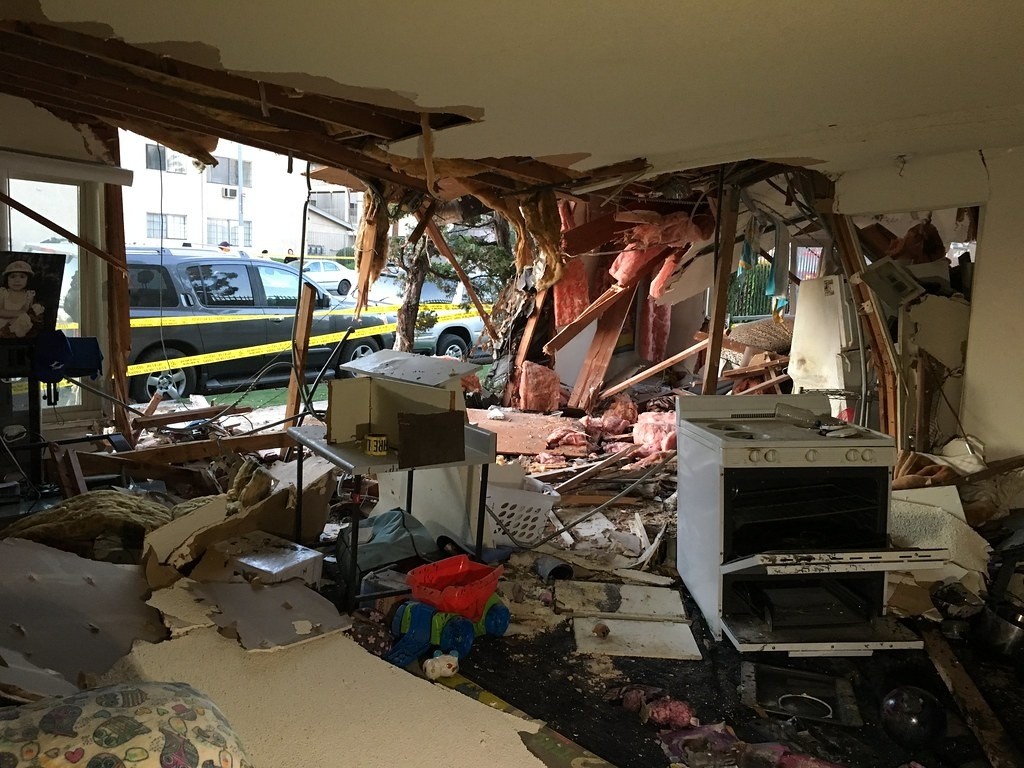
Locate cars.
[282,259,358,296]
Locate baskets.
[483,475,562,543]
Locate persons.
[0,261,42,339]
[262,250,272,261]
[218,242,233,252]
[284,249,298,264]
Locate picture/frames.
[0,251,67,340]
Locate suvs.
[23,241,392,407]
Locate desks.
[289,425,496,603]
